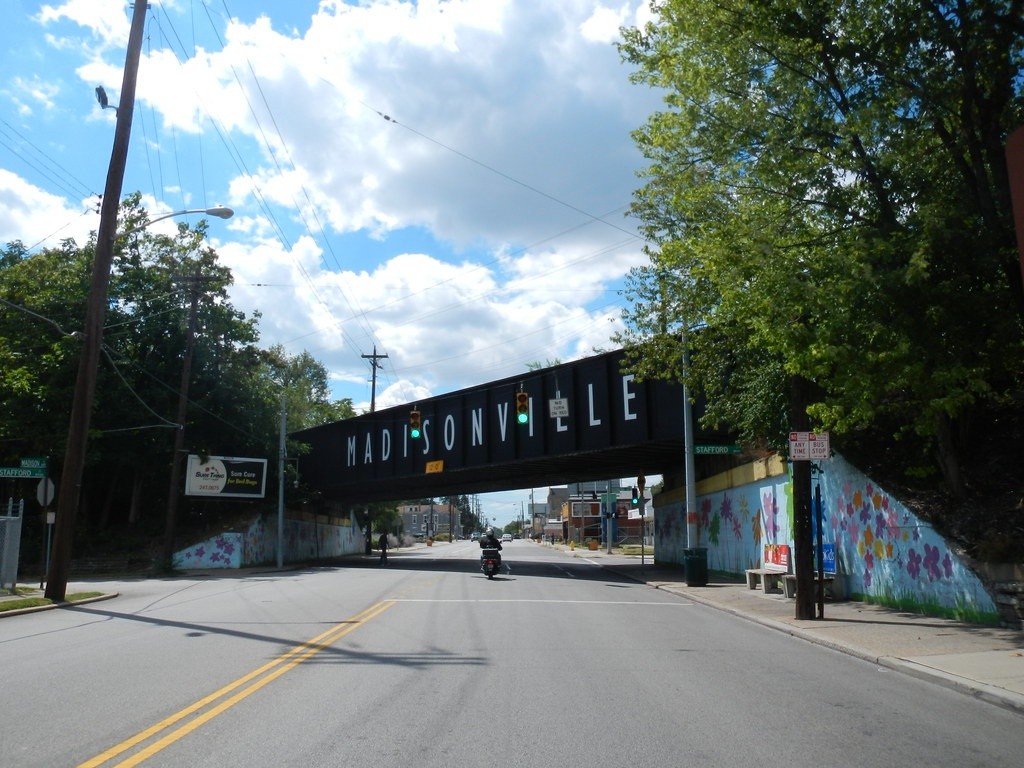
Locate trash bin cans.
[683,548,708,586]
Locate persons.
[480,526,502,570]
[551,532,554,544]
[379,530,390,562]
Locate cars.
[482,533,487,538]
[454,535,465,540]
[471,532,480,542]
[502,534,512,542]
[530,533,544,540]
[514,533,521,539]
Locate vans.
[414,533,427,543]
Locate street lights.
[512,503,525,539]
[43,205,234,602]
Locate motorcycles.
[480,540,502,578]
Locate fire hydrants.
[569,541,575,551]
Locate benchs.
[781,574,836,601]
[745,568,785,593]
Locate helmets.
[486,527,494,535]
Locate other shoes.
[480,563,485,572]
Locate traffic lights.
[518,393,528,425]
[408,410,421,439]
[632,488,639,509]
